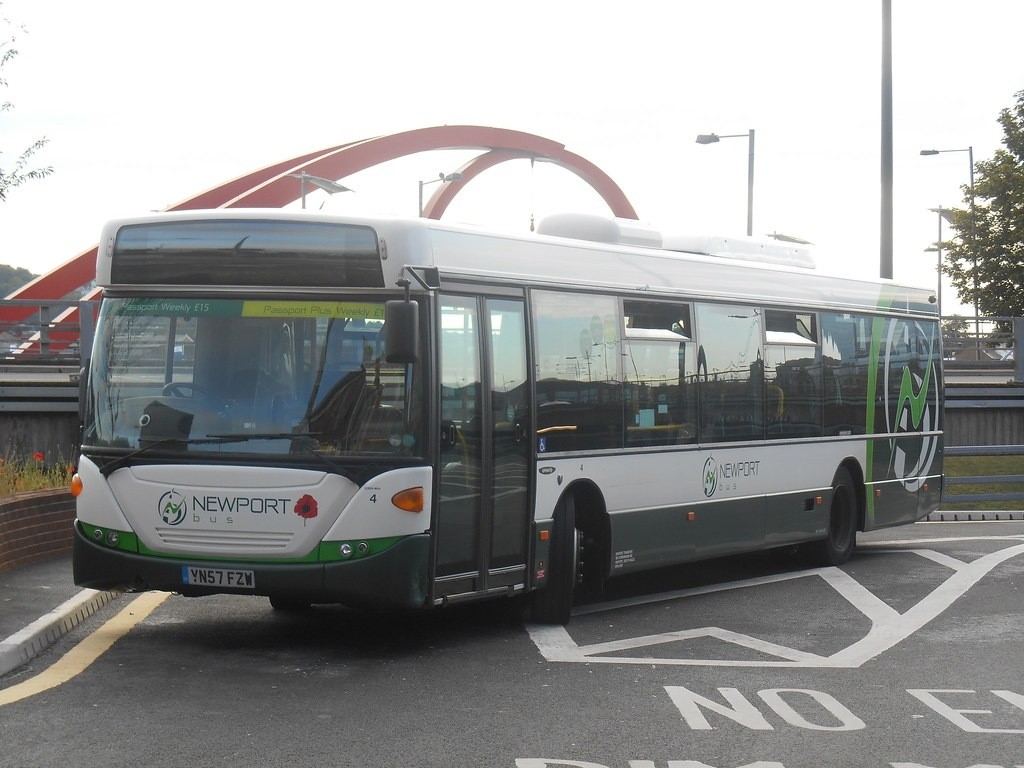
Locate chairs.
[765,366,841,421]
[286,360,366,447]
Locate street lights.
[918,146,981,361]
[696,128,754,237]
[418,171,463,217]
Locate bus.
[70,205,950,629]
